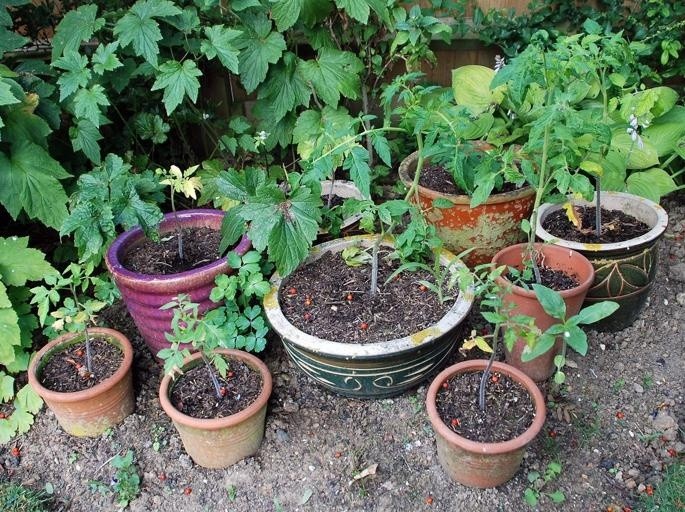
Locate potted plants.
[425,262,548,491]
[26,260,134,440]
[158,291,273,472]
[102,1,599,402]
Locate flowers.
[486,18,665,238]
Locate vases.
[533,190,671,333]
